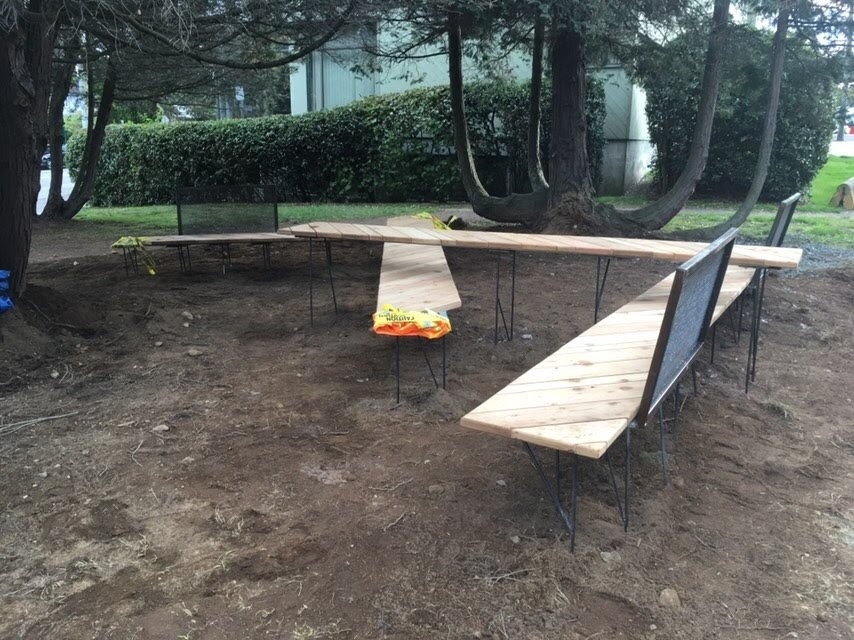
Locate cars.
[41,143,67,170]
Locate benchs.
[111,193,804,552]
